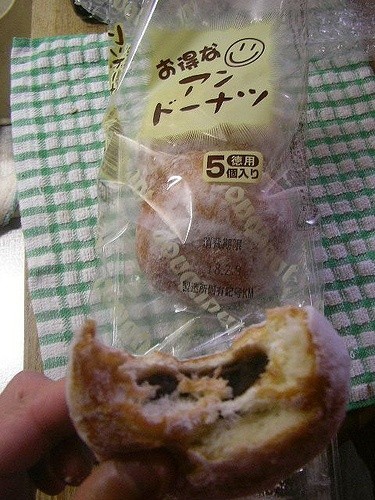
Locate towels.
[10,14,374,412]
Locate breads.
[135,154,295,313]
[61,302,354,494]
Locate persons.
[0,370,174,499]
